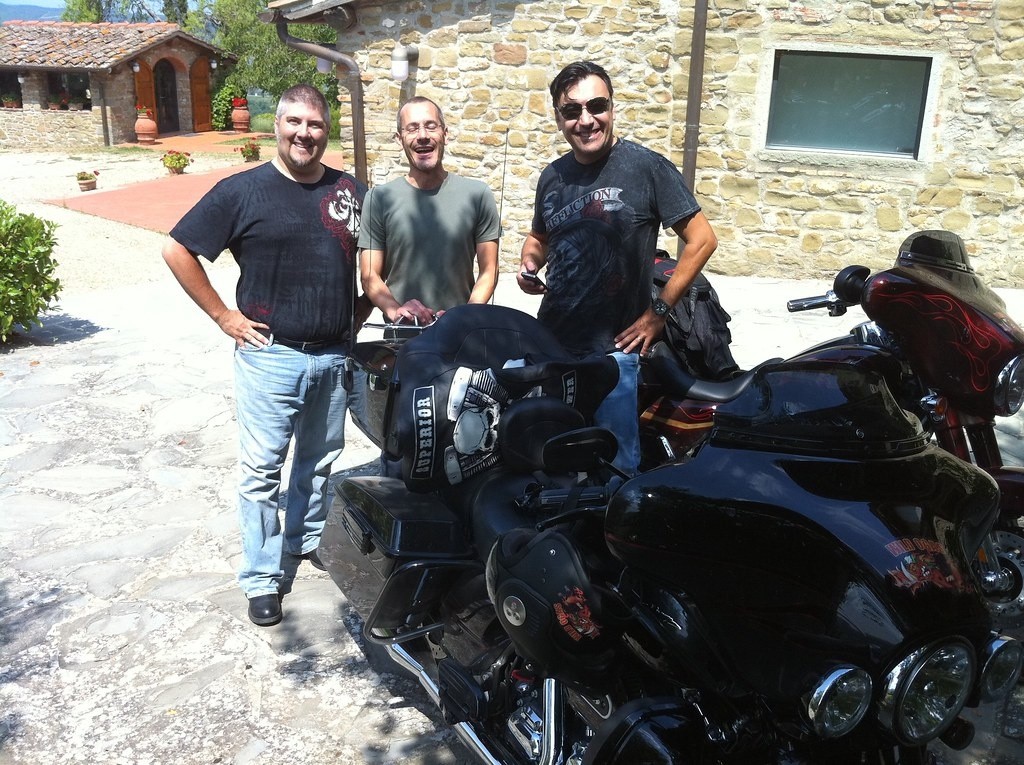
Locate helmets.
[485,528,636,671]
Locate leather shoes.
[292,546,327,570]
[248,594,282,625]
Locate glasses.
[555,95,612,120]
[400,122,443,134]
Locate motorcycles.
[316,230,1023,765]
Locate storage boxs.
[345,335,410,450]
[316,476,466,629]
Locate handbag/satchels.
[647,249,741,382]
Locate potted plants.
[66,96,84,110]
[0,91,22,108]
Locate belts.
[277,337,351,351]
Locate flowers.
[75,171,100,181]
[46,93,70,104]
[233,98,248,107]
[136,105,153,118]
[233,138,260,159]
[160,150,194,168]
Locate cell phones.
[521,272,549,292]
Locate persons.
[516,60,718,481]
[357,96,505,340]
[162,83,369,627]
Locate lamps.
[128,59,140,73]
[208,58,217,70]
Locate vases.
[48,102,62,110]
[231,106,250,134]
[135,113,158,145]
[246,154,259,162]
[167,167,184,174]
[77,180,96,191]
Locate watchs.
[651,298,671,319]
